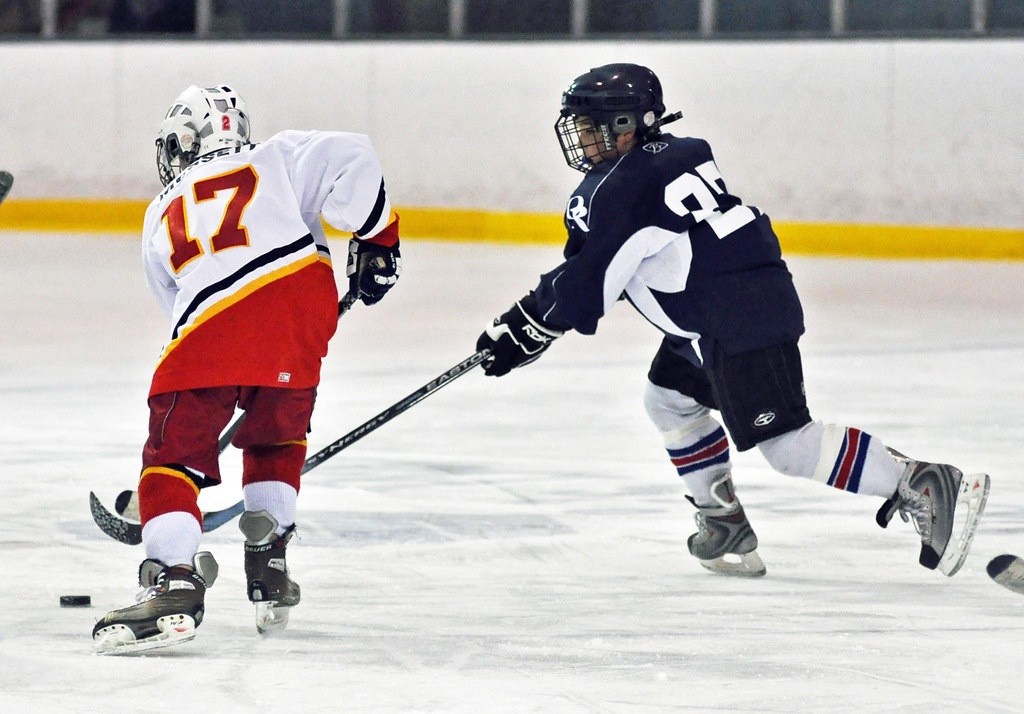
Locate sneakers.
[239,510,300,634]
[93,551,220,654]
[684,492,766,578]
[876,447,989,576]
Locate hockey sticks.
[112,347,493,532]
[985,553,1024,596]
[88,254,387,546]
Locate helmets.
[553,64,664,174]
[155,83,251,185]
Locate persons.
[91,83,402,652]
[476,63,990,578]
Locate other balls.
[59,595,92,609]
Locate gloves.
[477,292,564,377]
[345,238,402,305]
